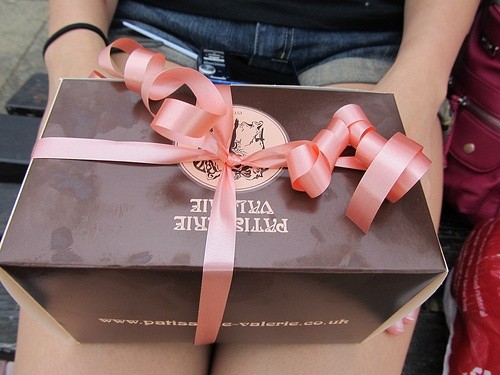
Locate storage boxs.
[0,76,450,346]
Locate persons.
[12,1,481,375]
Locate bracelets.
[41,22,108,58]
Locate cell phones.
[122,19,300,85]
